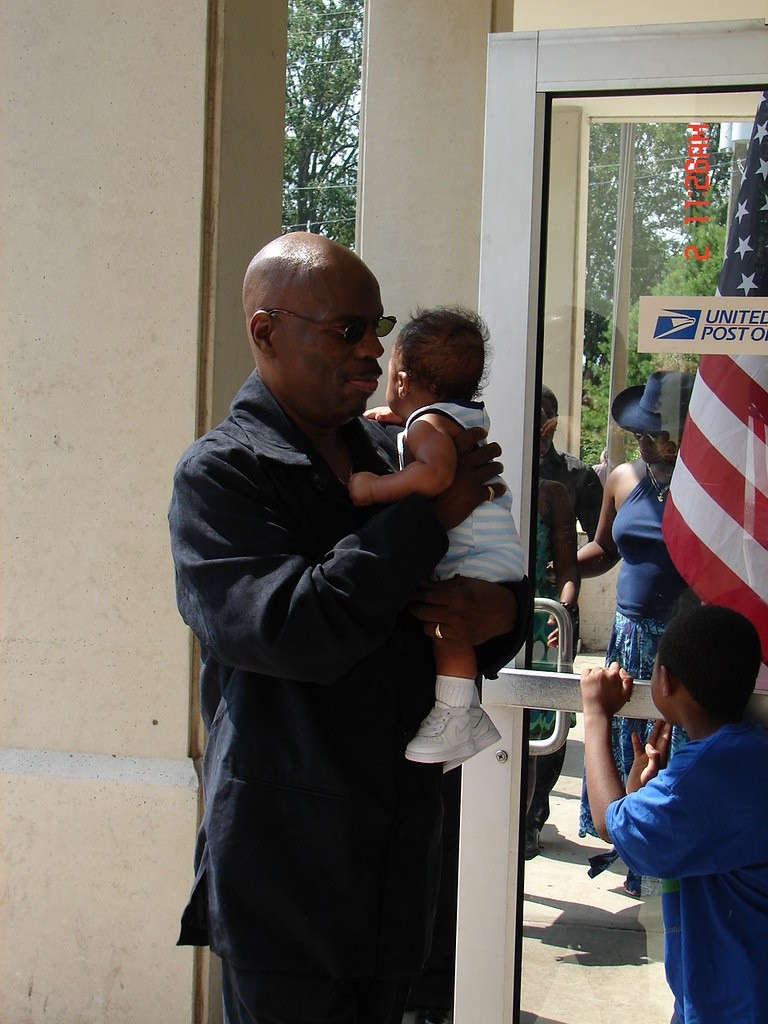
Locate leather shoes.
[526,802,549,860]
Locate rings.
[484,485,494,502]
[434,623,444,639]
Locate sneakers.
[405,707,475,763]
[442,706,502,773]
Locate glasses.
[266,308,396,344]
[636,432,660,441]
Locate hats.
[611,372,695,435]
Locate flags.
[661,91,767,666]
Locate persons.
[581,605,768,1024]
[349,310,524,772]
[522,371,693,895]
[169,233,534,1024]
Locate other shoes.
[625,876,643,896]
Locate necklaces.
[647,463,670,501]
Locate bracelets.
[559,602,578,624]
